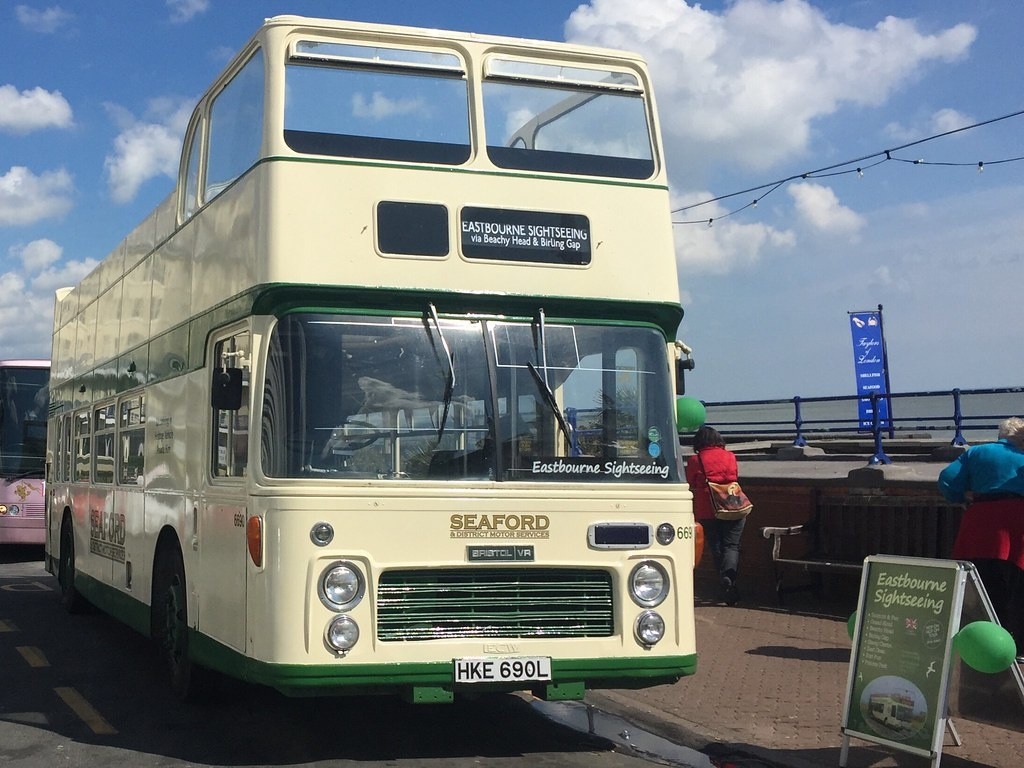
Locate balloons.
[953,620,1016,674]
[847,610,857,639]
[677,397,706,433]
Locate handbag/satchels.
[708,481,753,520]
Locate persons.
[685,426,746,608]
[939,417,1024,656]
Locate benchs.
[759,488,966,606]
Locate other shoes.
[720,570,737,597]
[719,589,744,606]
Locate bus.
[41,12,697,717]
[0,360,50,545]
